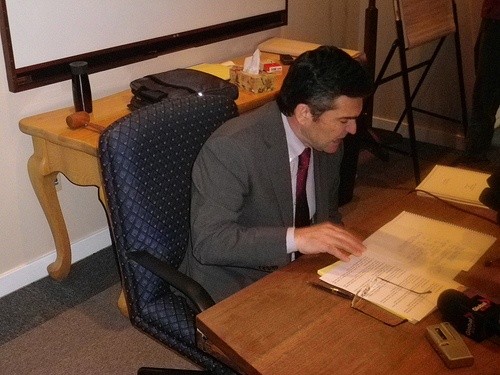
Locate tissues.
[228,48,275,94]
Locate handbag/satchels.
[127,67,239,112]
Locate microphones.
[436,288,500,344]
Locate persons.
[454,0,500,163]
[168,44,371,311]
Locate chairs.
[98,94,239,375]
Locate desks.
[19,48,361,318]
[196,166,500,375]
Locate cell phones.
[279,54,295,65]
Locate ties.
[294,147,311,261]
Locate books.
[415,164,493,210]
